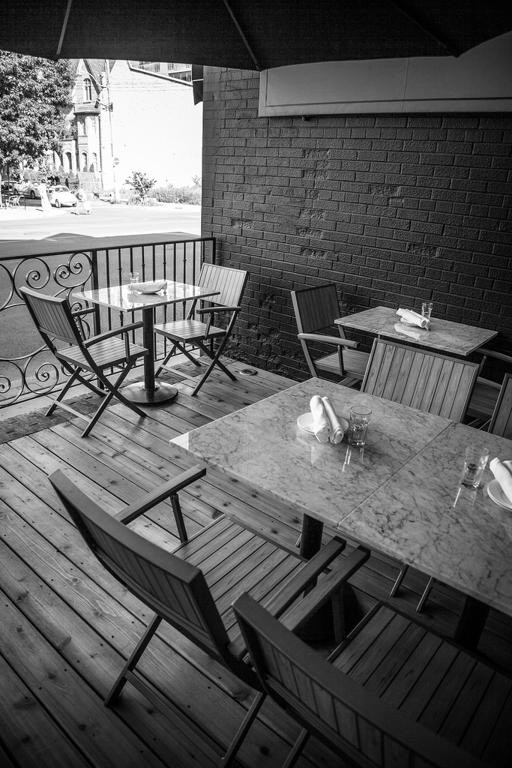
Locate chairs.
[290,283,512,439]
[230,543,512,766]
[48,463,345,768]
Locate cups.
[461,444,488,489]
[128,272,139,290]
[346,407,373,446]
[420,301,432,323]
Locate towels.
[489,457,512,503]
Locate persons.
[38,179,52,213]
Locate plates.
[297,412,348,436]
[486,478,512,510]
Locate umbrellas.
[0,0,511,72]
